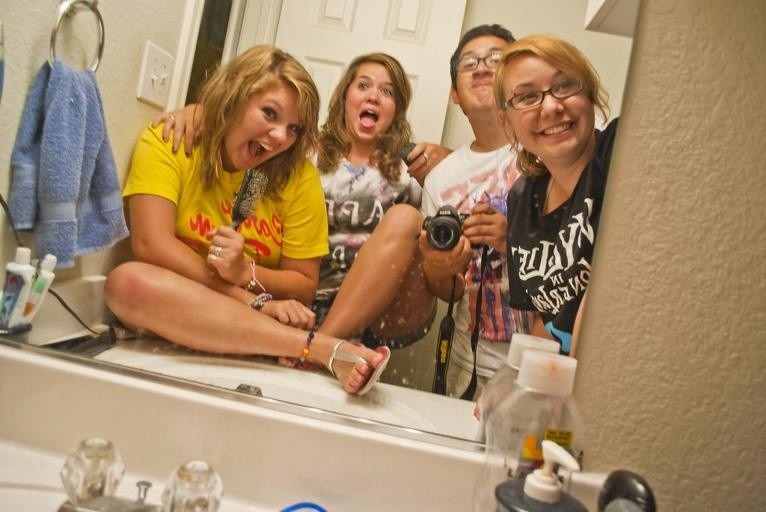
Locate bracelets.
[249,292,272,311]
[246,260,265,293]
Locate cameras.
[423,205,482,251]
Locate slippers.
[329,340,390,396]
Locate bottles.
[473,333,564,446]
[470,351,584,512]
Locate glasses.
[451,54,503,76]
[502,78,582,110]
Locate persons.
[418,24,540,403]
[474,34,620,423]
[105,43,392,398]
[152,53,454,370]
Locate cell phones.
[400,143,423,186]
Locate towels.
[5,58,131,272]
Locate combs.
[232,168,268,232]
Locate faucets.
[235,382,264,396]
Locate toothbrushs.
[24,253,57,316]
[22,270,56,325]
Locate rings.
[168,115,175,122]
[423,153,429,162]
[209,255,216,265]
[214,246,221,257]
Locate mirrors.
[0,0,648,456]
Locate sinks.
[1,482,68,510]
[147,362,435,433]
